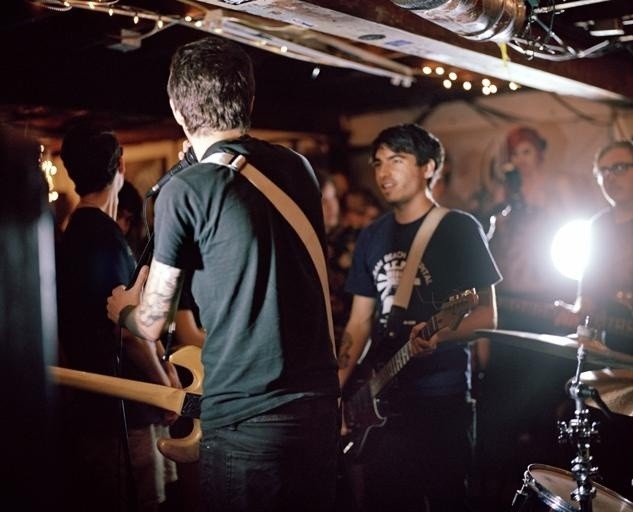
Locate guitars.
[496,291,633,338]
[49,344,205,463]
[338,288,480,466]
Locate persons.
[312,148,471,327]
[117,179,142,239]
[107,37,341,512]
[495,127,571,292]
[336,123,497,512]
[555,142,633,355]
[55,129,183,512]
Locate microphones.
[145,145,199,197]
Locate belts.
[244,395,338,422]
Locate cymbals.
[566,369,633,416]
[475,329,633,369]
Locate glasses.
[595,162,633,176]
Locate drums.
[510,465,633,512]
